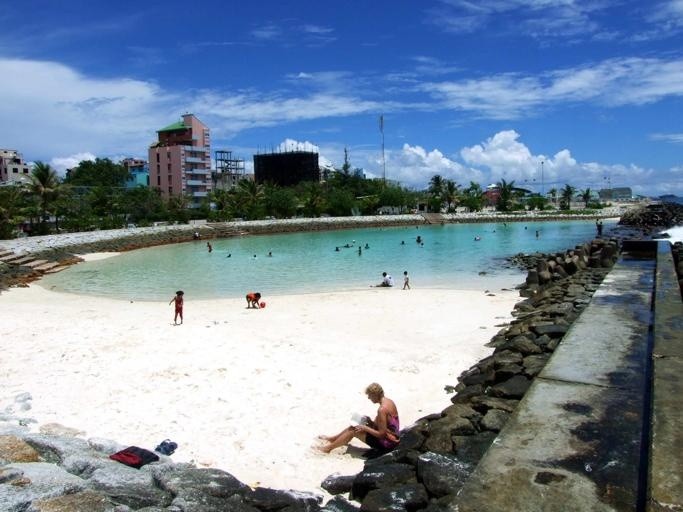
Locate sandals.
[156,439,178,454]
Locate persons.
[246,292,261,308]
[402,271,411,289]
[376,272,394,287]
[335,235,423,255]
[207,242,213,252]
[316,382,399,453]
[169,291,184,324]
[596,218,604,235]
[194,232,199,240]
